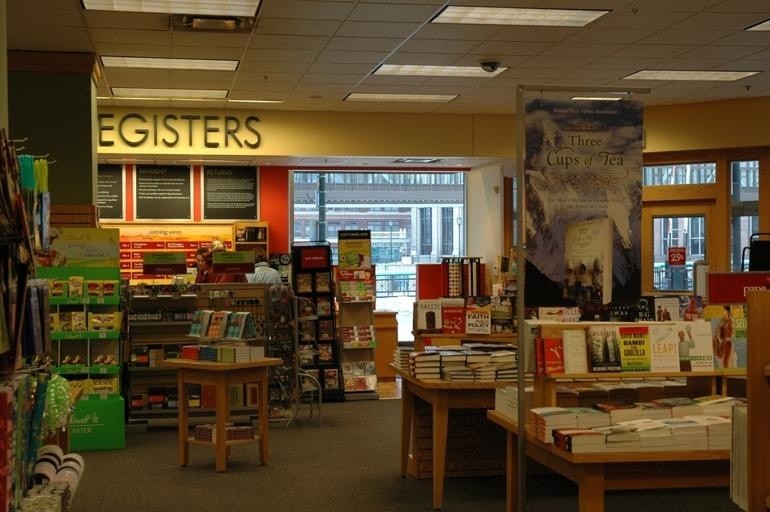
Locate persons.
[681,297,700,321]
[656,306,663,321]
[565,258,604,306]
[662,308,671,321]
[678,325,696,371]
[716,304,736,368]
[190,240,282,283]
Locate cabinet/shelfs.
[33,270,275,475]
[231,221,270,262]
[292,241,378,404]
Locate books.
[552,428,606,454]
[617,419,673,451]
[616,327,650,371]
[703,304,747,368]
[676,321,714,371]
[296,270,339,391]
[589,326,622,372]
[392,341,415,371]
[495,384,519,425]
[439,297,465,333]
[729,405,748,510]
[409,343,518,382]
[693,394,736,417]
[562,329,588,375]
[588,424,641,453]
[491,250,518,315]
[417,299,442,330]
[652,397,703,418]
[678,295,704,321]
[416,257,493,301]
[648,326,680,372]
[653,418,708,450]
[567,408,610,429]
[591,400,641,425]
[629,401,672,419]
[177,309,265,443]
[681,416,731,449]
[523,302,634,335]
[465,295,492,333]
[491,297,515,333]
[630,296,679,321]
[525,405,577,443]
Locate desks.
[386,332,748,512]
[154,352,284,472]
